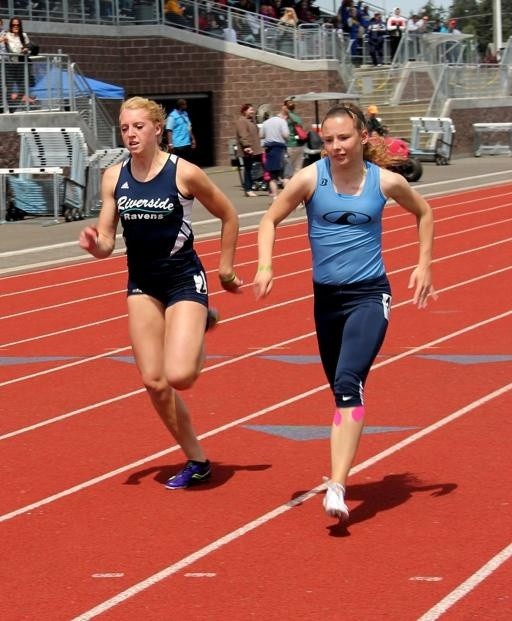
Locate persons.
[237,100,304,197]
[79,96,245,491]
[165,98,195,159]
[3,16,31,112]
[0,1,462,66]
[253,102,439,521]
[366,105,388,137]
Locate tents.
[6,62,124,106]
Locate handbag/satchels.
[292,125,308,140]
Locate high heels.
[245,191,257,197]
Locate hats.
[367,104,380,116]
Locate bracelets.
[222,274,236,283]
[258,264,273,273]
[168,144,173,146]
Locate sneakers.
[321,476,349,519]
[165,457,213,491]
